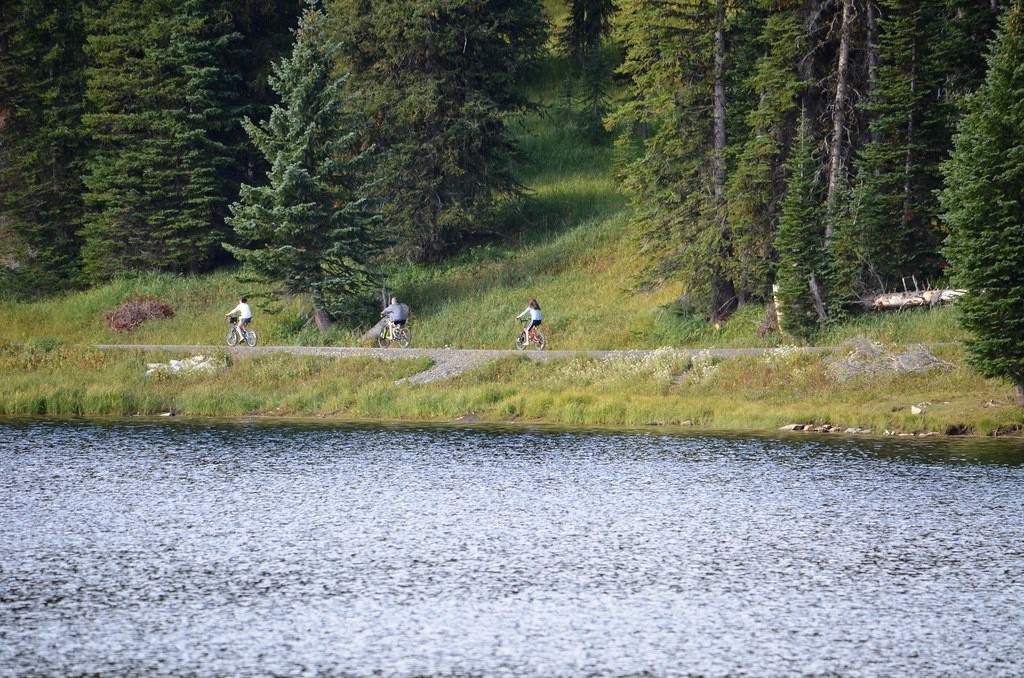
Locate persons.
[381,297,407,341]
[226,296,252,343]
[517,299,541,346]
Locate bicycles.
[516,318,545,350]
[224,315,258,347]
[377,314,412,349]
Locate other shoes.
[240,337,245,341]
[523,342,530,346]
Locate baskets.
[228,317,237,323]
